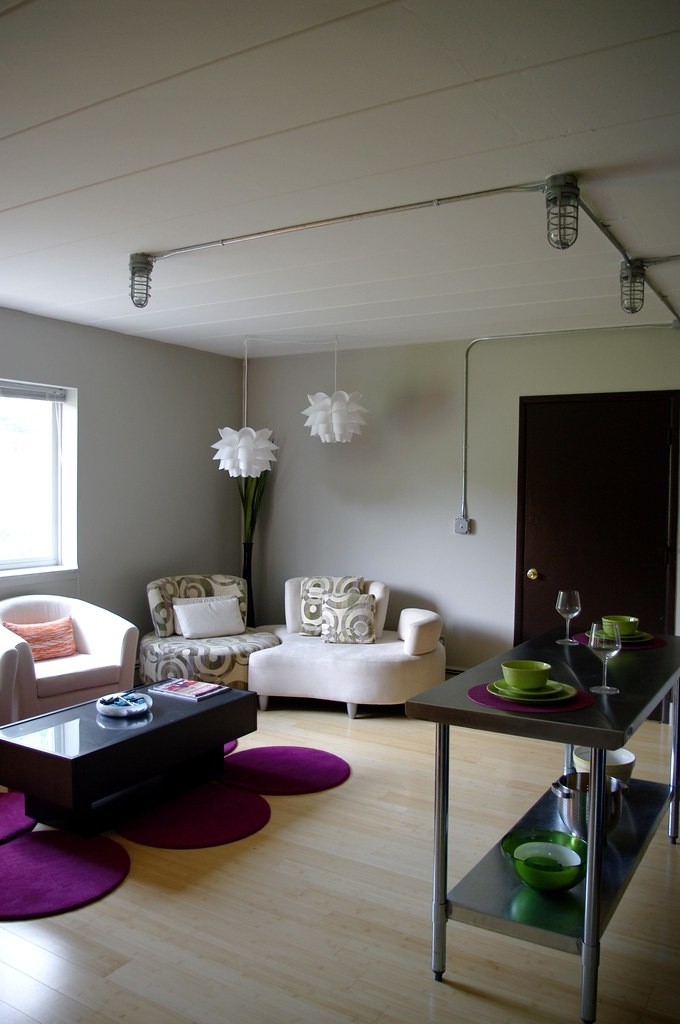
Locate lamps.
[300,337,367,443]
[129,253,153,308]
[545,173,580,249]
[210,338,279,479]
[620,258,645,314]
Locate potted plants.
[235,471,268,628]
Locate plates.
[572,631,672,649]
[467,681,594,712]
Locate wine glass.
[555,590,581,645]
[590,623,621,693]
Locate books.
[148,677,231,703]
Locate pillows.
[321,592,376,644]
[300,575,364,636]
[3,616,78,661]
[172,595,245,639]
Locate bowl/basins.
[501,661,552,688]
[499,883,583,938]
[602,616,639,636]
[500,828,587,892]
[96,692,154,718]
[573,746,636,782]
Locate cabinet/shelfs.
[405,623,680,1022]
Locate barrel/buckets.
[552,772,627,842]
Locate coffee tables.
[0,678,257,835]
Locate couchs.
[139,572,280,718]
[0,594,139,726]
[247,578,447,719]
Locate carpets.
[0,742,350,921]
